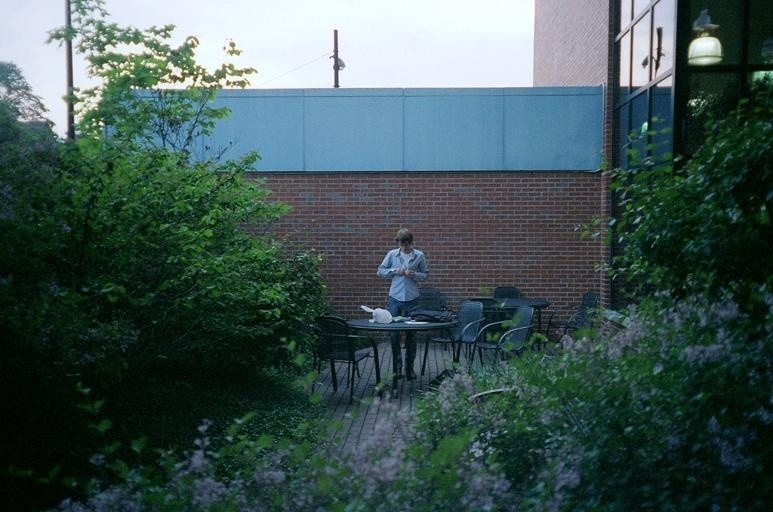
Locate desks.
[347,318,459,399]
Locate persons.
[375,228,429,380]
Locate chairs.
[311,316,370,405]
[416,286,598,372]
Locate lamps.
[687,9,724,66]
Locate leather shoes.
[397,369,416,379]
